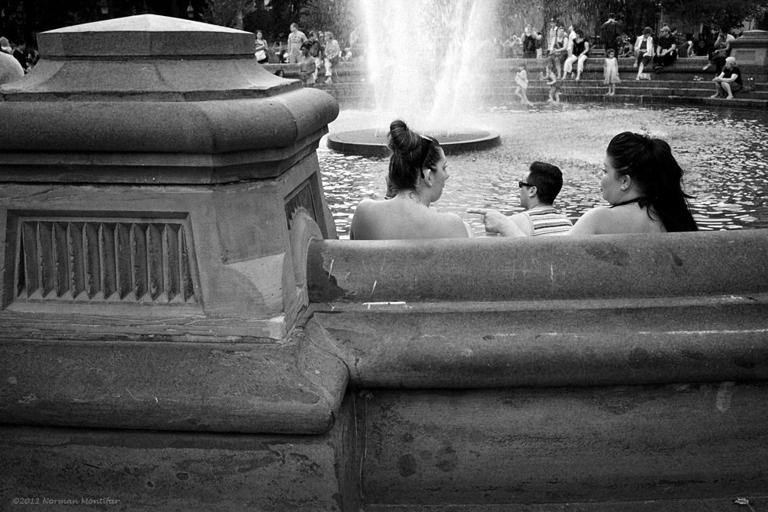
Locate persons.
[497,11,742,106]
[255,22,363,84]
[466,130,699,233]
[497,161,573,238]
[349,120,473,239]
[1,37,38,83]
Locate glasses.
[518,180,528,187]
